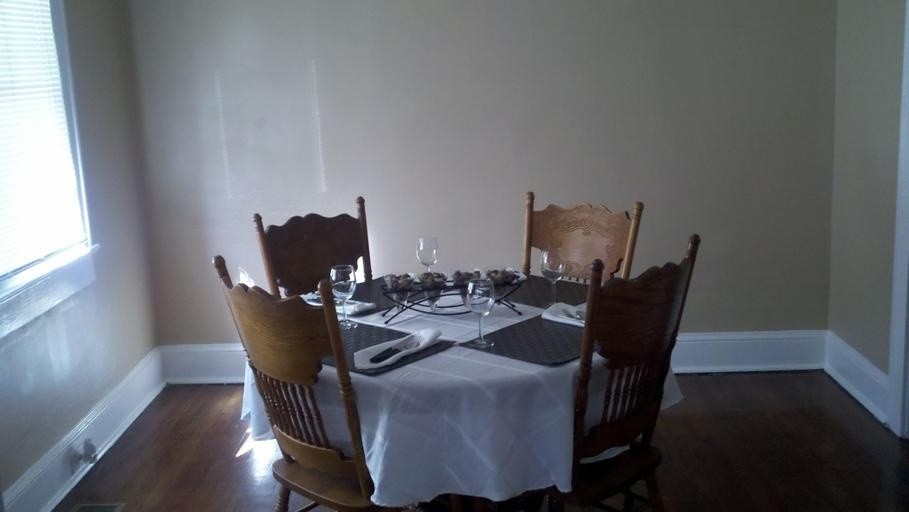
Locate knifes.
[369,334,416,363]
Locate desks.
[275,274,670,512]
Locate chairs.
[515,232,703,512]
[251,196,373,302]
[521,190,645,283]
[211,255,463,512]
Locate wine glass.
[330,264,358,330]
[414,234,440,274]
[465,278,495,349]
[540,250,567,304]
